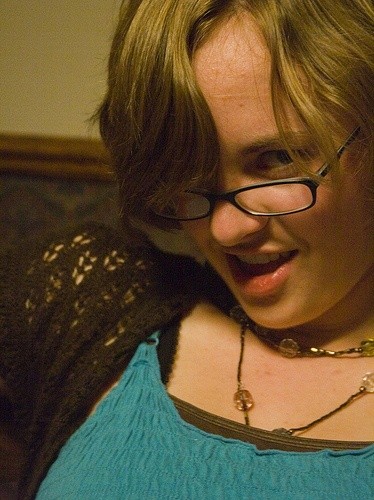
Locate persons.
[0,0,374,500]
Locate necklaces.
[230,302,374,440]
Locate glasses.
[142,115,373,221]
